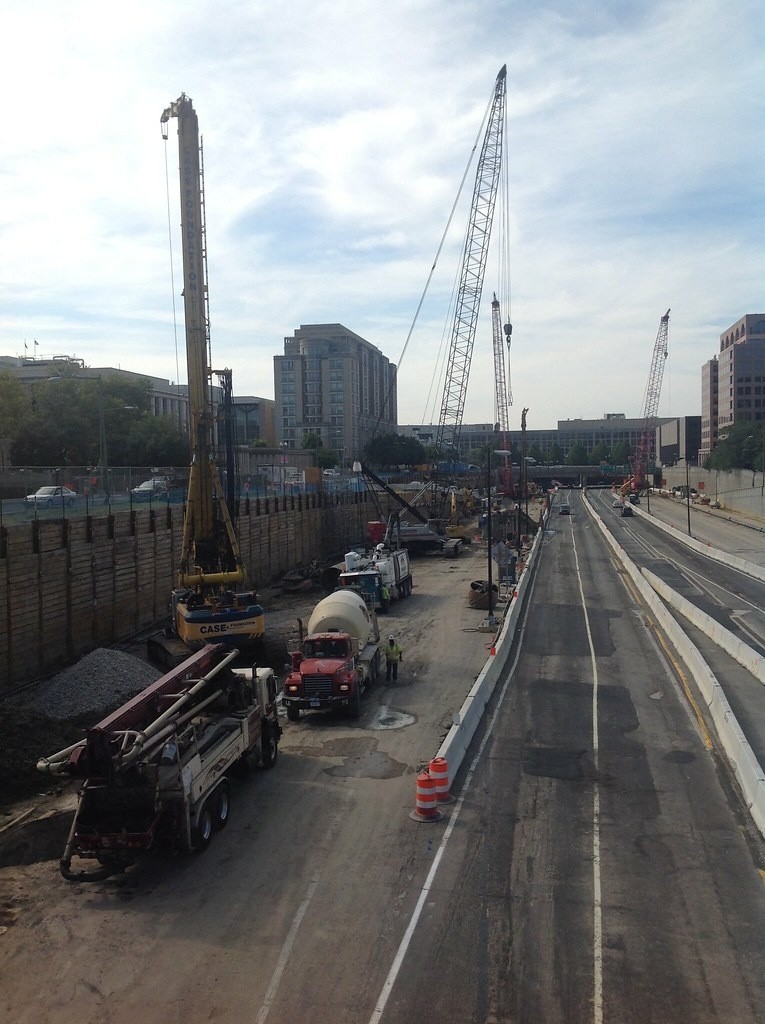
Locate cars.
[129,480,176,501]
[620,507,633,517]
[23,485,77,509]
[629,494,640,504]
[559,505,570,515]
[612,500,624,508]
[242,466,341,497]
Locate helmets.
[389,635,394,640]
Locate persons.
[384,634,403,685]
[379,584,390,614]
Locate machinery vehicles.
[160,90,269,649]
[282,589,380,725]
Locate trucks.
[336,546,413,613]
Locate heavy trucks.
[66,650,285,861]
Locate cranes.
[521,407,531,503]
[490,292,515,502]
[344,61,512,558]
[621,308,672,493]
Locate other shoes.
[385,680,399,685]
